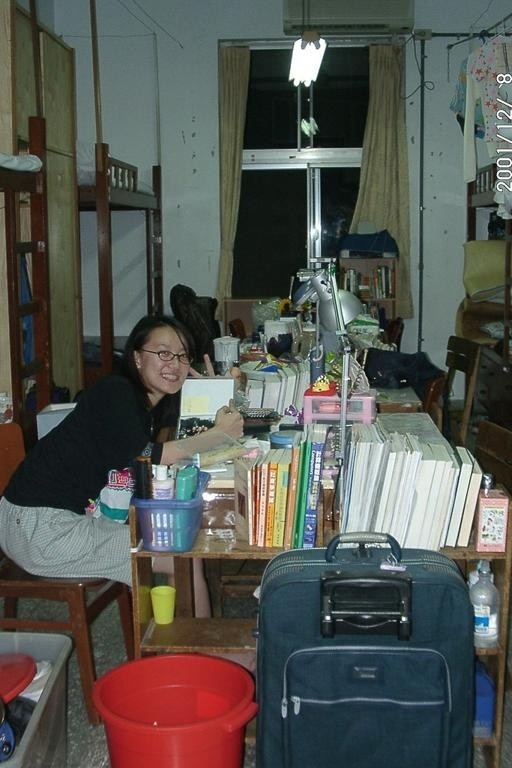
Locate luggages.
[253,532,476,765]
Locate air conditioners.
[283,0,415,36]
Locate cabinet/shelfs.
[0,0,86,398]
[339,256,399,321]
[127,499,512,768]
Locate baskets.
[128,470,211,554]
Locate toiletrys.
[151,464,174,548]
[175,466,198,550]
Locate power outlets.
[414,29,432,40]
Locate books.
[208,417,484,553]
[238,359,312,416]
[174,375,238,440]
[338,263,395,300]
[262,312,306,343]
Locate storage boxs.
[0,632,72,768]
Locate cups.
[150,586,176,625]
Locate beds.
[465,163,512,429]
[77,143,164,403]
[0,117,51,453]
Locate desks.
[173,410,456,619]
[373,383,423,413]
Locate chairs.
[423,368,447,413]
[473,419,512,498]
[441,335,482,448]
[0,423,135,727]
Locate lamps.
[295,115,320,137]
[306,255,364,473]
[288,0,328,88]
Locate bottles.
[469,566,500,648]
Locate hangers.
[479,25,512,47]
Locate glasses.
[142,347,194,365]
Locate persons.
[0,311,258,675]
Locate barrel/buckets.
[94,653,259,767]
